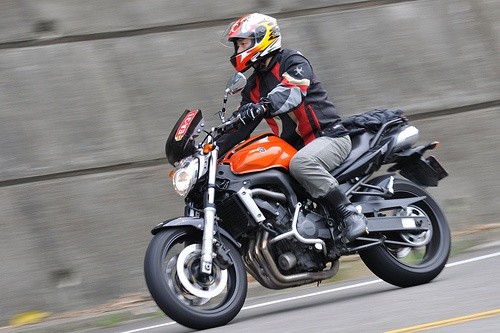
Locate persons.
[216,12,367,242]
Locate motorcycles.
[142,71,453,331]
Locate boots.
[320,185,368,238]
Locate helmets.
[217,11,282,72]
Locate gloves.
[231,103,266,128]
